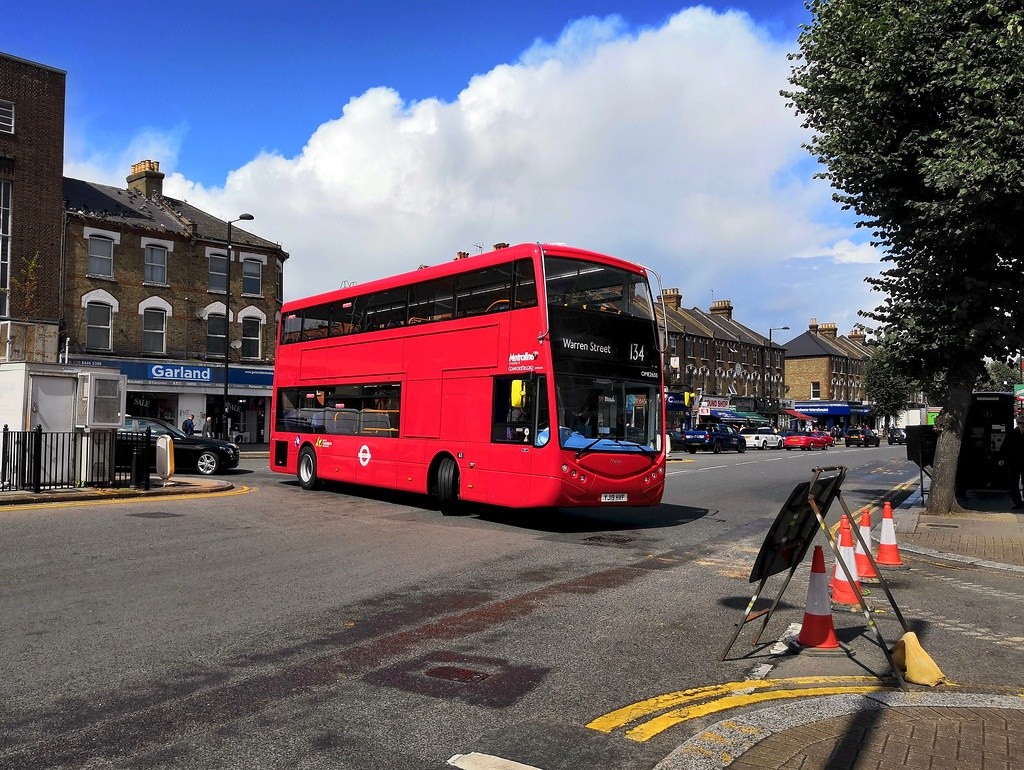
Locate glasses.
[1017,422,1024,425]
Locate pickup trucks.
[681,423,747,454]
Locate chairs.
[723,429,728,433]
[282,318,430,344]
[566,406,591,438]
[285,409,387,436]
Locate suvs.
[844,428,881,448]
[887,428,908,445]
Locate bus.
[269,242,677,517]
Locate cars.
[813,431,836,447]
[785,432,827,451]
[739,428,784,450]
[115,416,241,475]
[655,430,690,454]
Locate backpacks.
[182,419,190,431]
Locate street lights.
[224,212,254,442]
[769,326,791,427]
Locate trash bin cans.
[130,447,146,489]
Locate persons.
[182,414,196,436]
[202,416,213,438]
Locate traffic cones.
[796,545,841,649]
[854,509,877,577]
[832,525,863,605]
[828,514,850,589]
[874,501,903,565]
